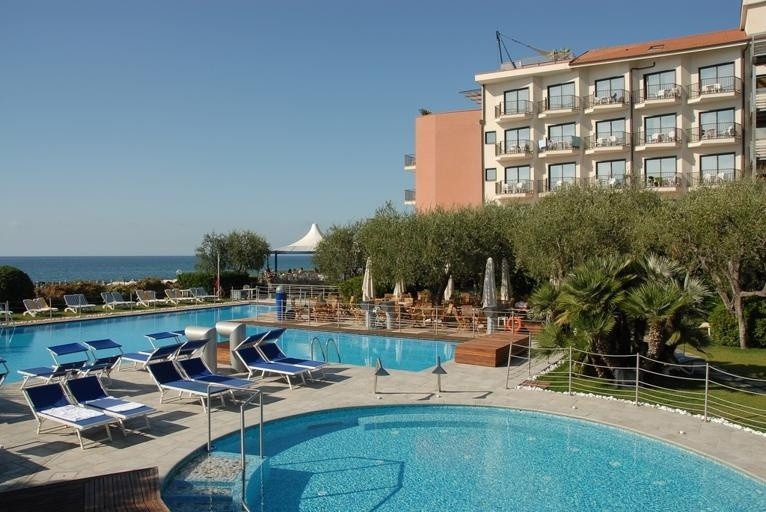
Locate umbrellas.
[362,257,374,302]
[499,256,513,300]
[483,257,497,309]
[444,275,453,302]
[393,277,405,331]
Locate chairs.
[22,286,219,318]
[23,382,119,452]
[258,328,325,383]
[18,343,90,390]
[177,356,257,404]
[147,361,230,413]
[137,329,188,356]
[52,338,122,377]
[122,332,176,370]
[65,375,159,439]
[235,332,309,387]
